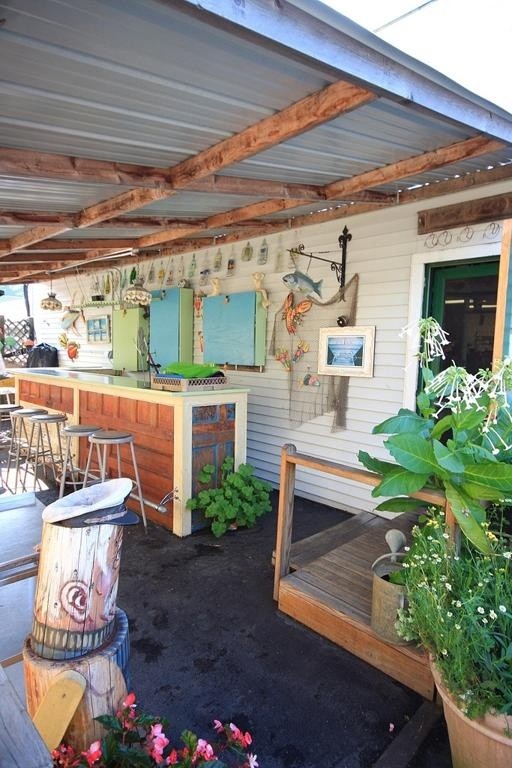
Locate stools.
[0,403,149,536]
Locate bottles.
[89,238,268,294]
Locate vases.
[425,651,509,766]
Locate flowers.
[43,691,261,767]
[405,505,512,722]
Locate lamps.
[38,270,62,311]
[122,260,152,307]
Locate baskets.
[150,372,229,392]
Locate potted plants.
[184,453,273,537]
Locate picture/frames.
[84,315,111,345]
[318,325,376,379]
